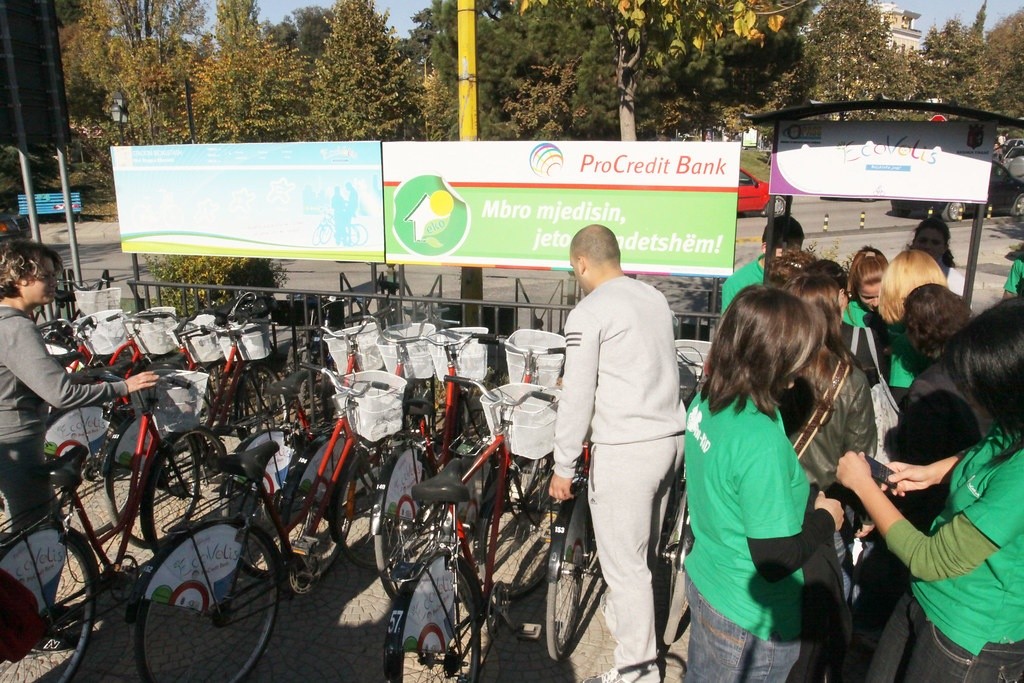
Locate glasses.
[844,291,855,298]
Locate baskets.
[427,327,489,383]
[74,287,121,315]
[333,369,407,442]
[505,329,566,388]
[130,369,210,440]
[165,313,271,363]
[479,382,562,460]
[322,322,437,379]
[124,306,178,355]
[70,309,129,353]
[674,339,714,401]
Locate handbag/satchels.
[850,324,903,465]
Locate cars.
[888,159,1024,220]
[735,169,792,215]
[993,137,1023,184]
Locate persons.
[548,224,686,683]
[0,241,160,653]
[685,217,1024,683]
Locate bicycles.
[0,270,719,683]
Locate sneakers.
[582,661,661,683]
[600,593,620,643]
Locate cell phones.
[864,454,897,489]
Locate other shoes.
[26,603,79,654]
